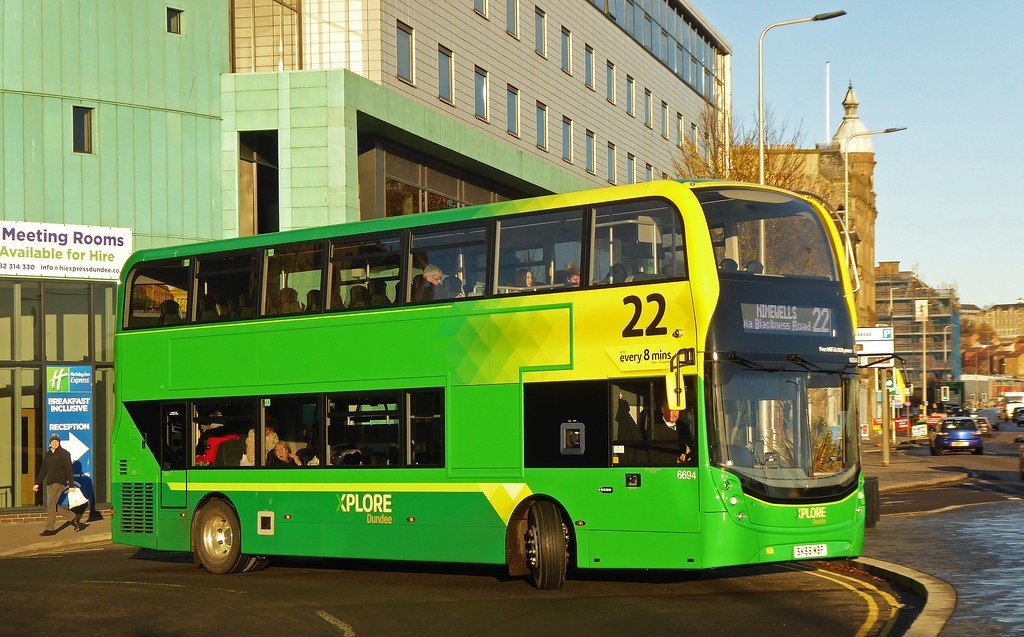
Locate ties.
[670,425,675,431]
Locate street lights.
[943,323,959,363]
[758,8,848,280]
[889,286,910,309]
[845,126,909,269]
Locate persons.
[566,273,580,285]
[32,436,81,536]
[414,264,447,302]
[649,400,693,464]
[196,411,303,466]
[512,269,533,287]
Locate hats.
[50,433,60,443]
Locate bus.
[108,176,916,592]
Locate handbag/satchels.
[68,488,89,509]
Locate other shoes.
[39,529,56,536]
[72,515,81,531]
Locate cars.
[978,409,1000,431]
[1011,406,1024,426]
[974,417,994,438]
[929,416,985,456]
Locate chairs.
[201,430,444,469]
[160,254,763,324]
